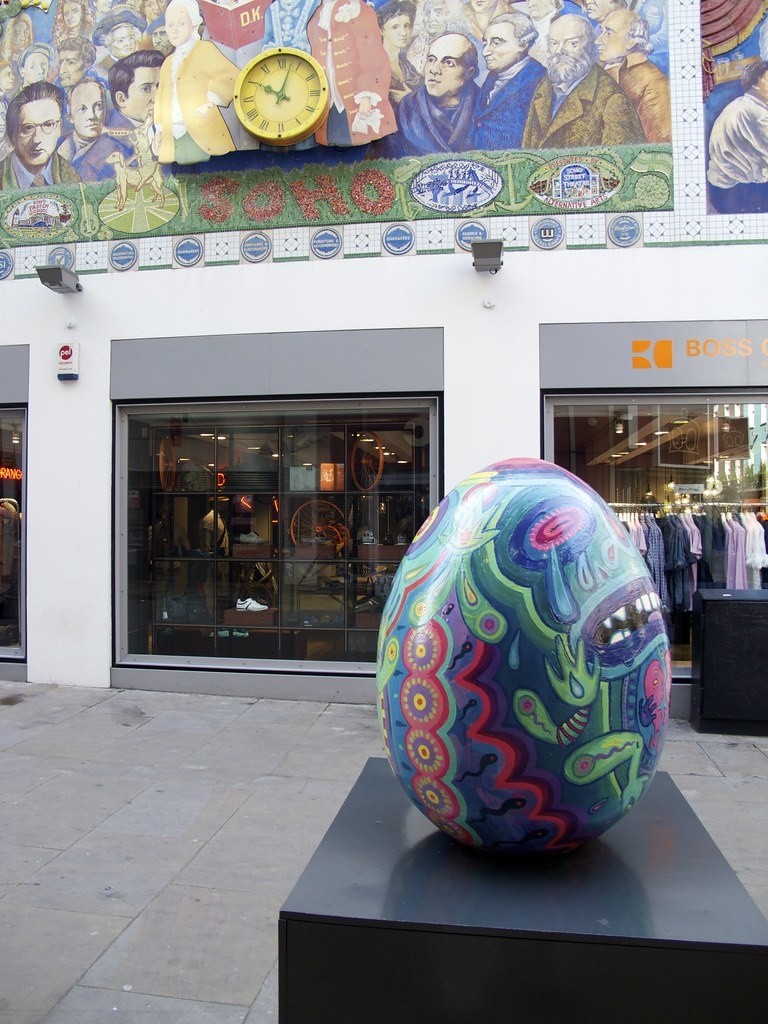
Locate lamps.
[35,265,83,294]
[11,428,19,443]
[721,416,731,433]
[471,240,505,274]
[614,418,624,434]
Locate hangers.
[612,504,766,523]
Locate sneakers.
[287,613,343,626]
[236,598,268,612]
[362,531,377,545]
[392,533,408,546]
[240,531,270,545]
[299,531,331,544]
[352,589,388,614]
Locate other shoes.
[158,545,188,559]
[181,547,214,559]
[0,624,20,645]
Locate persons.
[200,508,230,627]
[641,491,660,513]
[145,502,191,655]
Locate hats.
[208,497,229,502]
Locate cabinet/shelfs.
[130,423,416,657]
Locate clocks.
[234,47,331,146]
